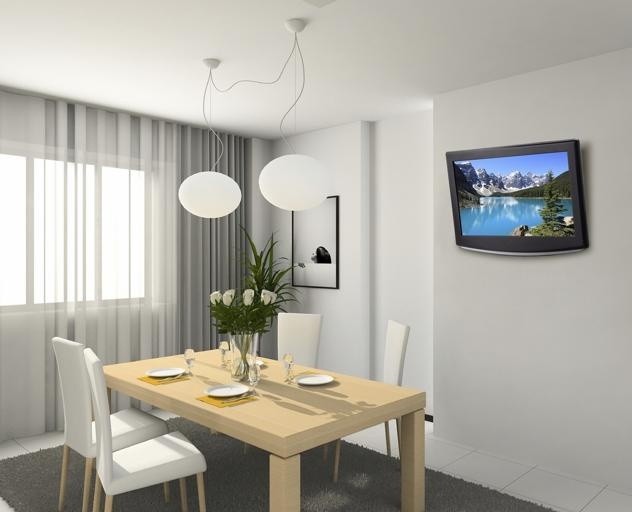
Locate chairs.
[321,318,411,483]
[82,346,208,512]
[242,310,329,458]
[51,336,170,512]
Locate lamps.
[173,17,335,222]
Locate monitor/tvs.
[445,139,589,257]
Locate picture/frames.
[290,195,340,290]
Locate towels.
[299,367,323,380]
[196,394,260,410]
[136,375,191,387]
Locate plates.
[295,375,333,387]
[145,368,185,378]
[204,384,249,399]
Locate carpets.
[0,416,553,512]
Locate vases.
[226,335,263,384]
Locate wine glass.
[184,349,195,377]
[219,341,229,368]
[249,363,260,397]
[283,352,293,383]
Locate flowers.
[205,224,304,382]
